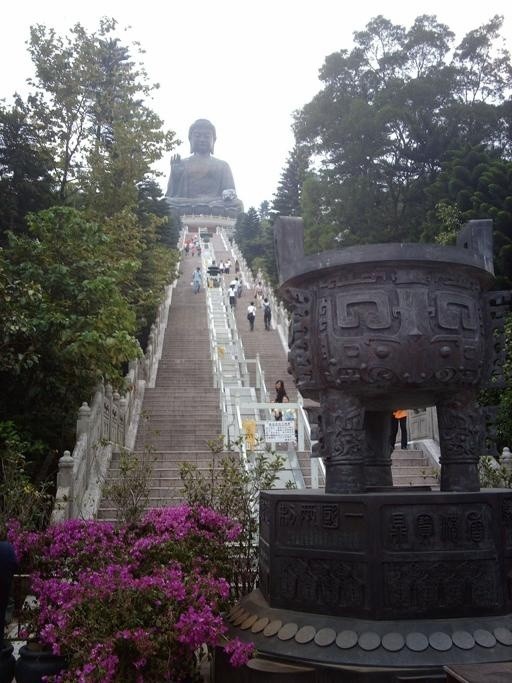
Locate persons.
[253,280,264,308]
[259,295,272,331]
[274,379,286,403]
[390,410,409,450]
[270,395,289,421]
[247,300,257,332]
[219,257,240,274]
[163,118,247,212]
[190,266,202,295]
[228,276,244,311]
[184,232,201,257]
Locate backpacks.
[263,303,271,316]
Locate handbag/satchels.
[247,312,254,320]
